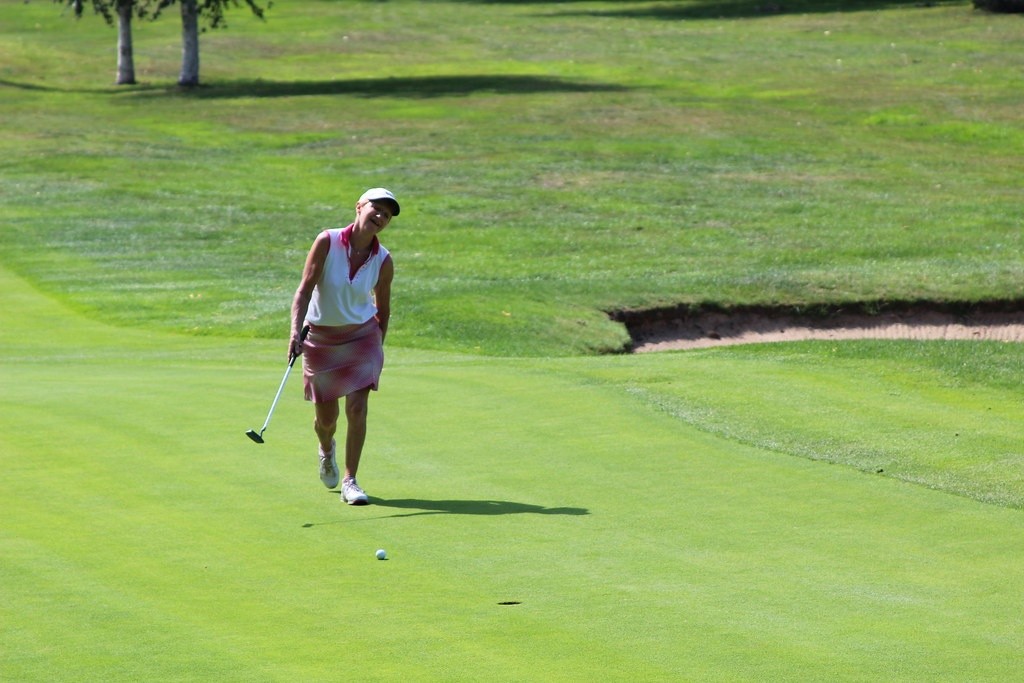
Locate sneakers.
[318,437,340,489]
[339,477,370,506]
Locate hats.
[358,187,401,217]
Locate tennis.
[376,549,386,560]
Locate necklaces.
[357,250,360,256]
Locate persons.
[288,188,400,505]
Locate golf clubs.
[244,324,310,444]
